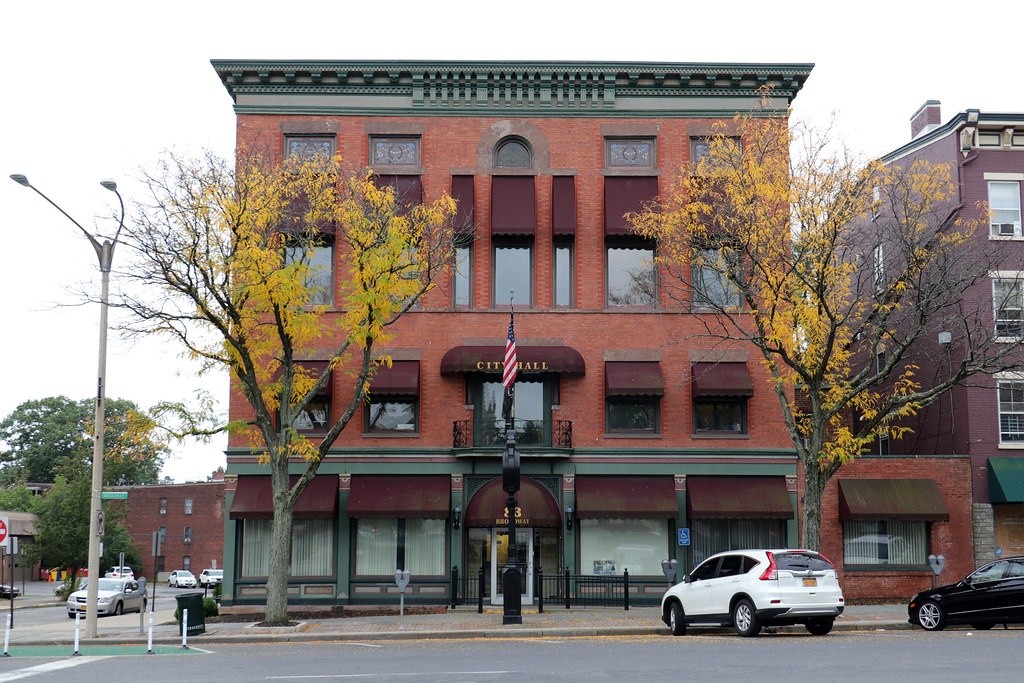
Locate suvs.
[198,569,223,589]
[105,566,135,579]
[660,547,845,637]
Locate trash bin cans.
[174,592,207,637]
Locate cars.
[167,570,197,589]
[55,577,88,597]
[66,577,147,618]
[908,556,1024,631]
[0,584,22,598]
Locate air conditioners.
[186,510,191,514]
[160,510,165,514]
[996,223,1015,236]
[185,538,190,543]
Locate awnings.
[574,475,679,521]
[986,457,1024,503]
[837,479,950,523]
[347,476,451,520]
[229,476,339,520]
[685,475,794,520]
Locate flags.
[502,308,517,390]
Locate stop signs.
[0,517,9,547]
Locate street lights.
[10,173,126,637]
